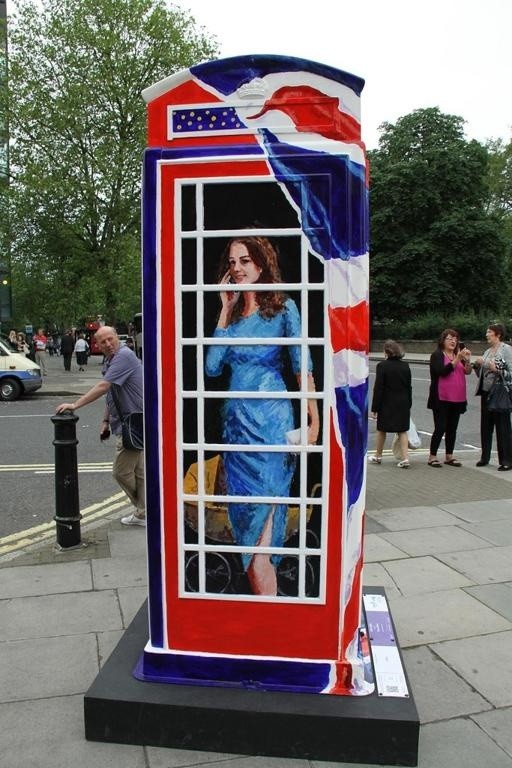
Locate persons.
[9,326,90,376]
[427,329,471,468]
[367,339,411,468]
[473,324,511,471]
[57,326,144,527]
[205,228,319,595]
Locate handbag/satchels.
[121,412,143,451]
[392,434,404,460]
[487,383,512,411]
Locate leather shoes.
[498,465,512,471]
[477,461,488,466]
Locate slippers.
[428,459,442,467]
[445,458,462,467]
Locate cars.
[0,338,42,401]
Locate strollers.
[183,454,322,596]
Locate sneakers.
[121,513,147,527]
[398,459,410,467]
[368,455,382,464]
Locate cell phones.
[459,342,465,351]
[101,430,110,441]
